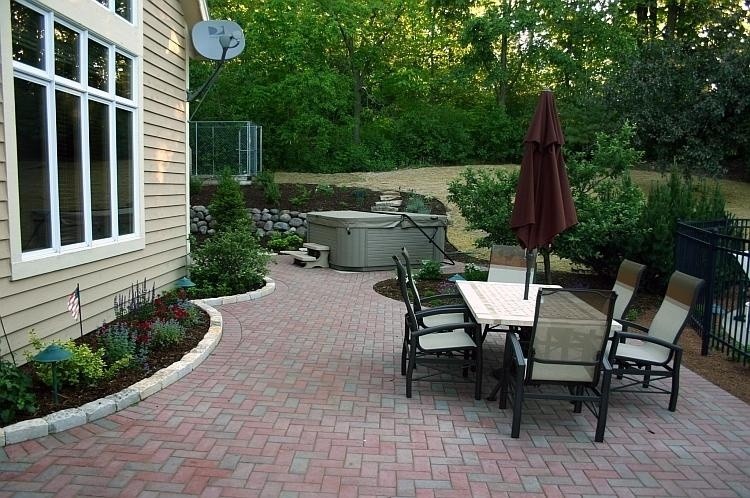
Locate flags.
[67,285,80,323]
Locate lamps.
[32,342,72,400]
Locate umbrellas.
[510,88,581,302]
